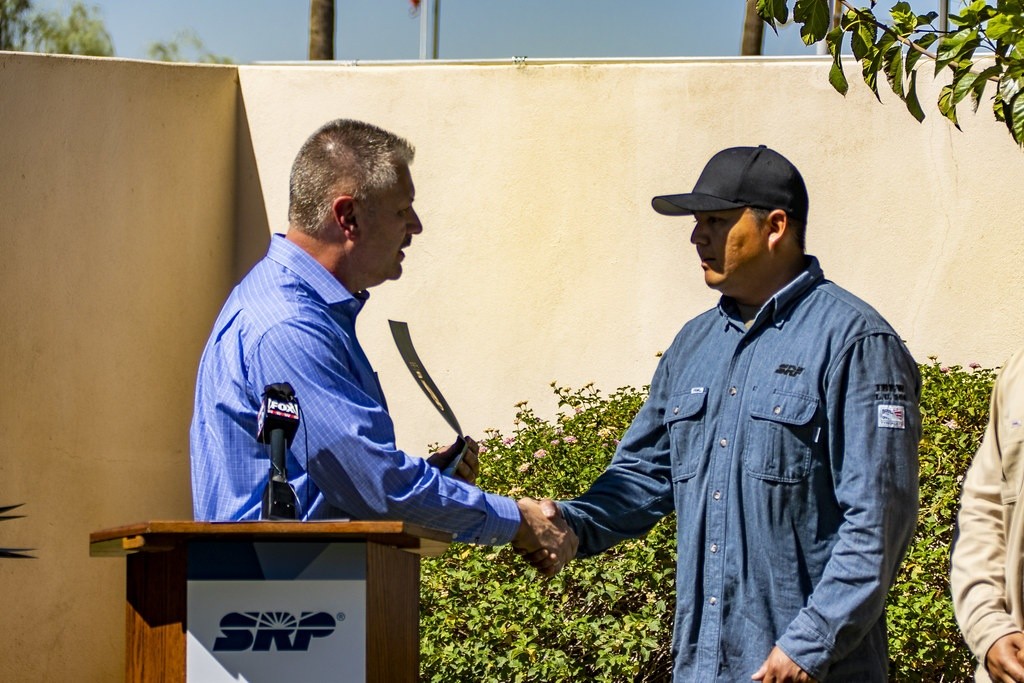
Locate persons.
[188,119,582,579]
[949,344,1024,682]
[512,141,921,681]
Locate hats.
[652,145,814,223]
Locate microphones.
[255,382,300,522]
